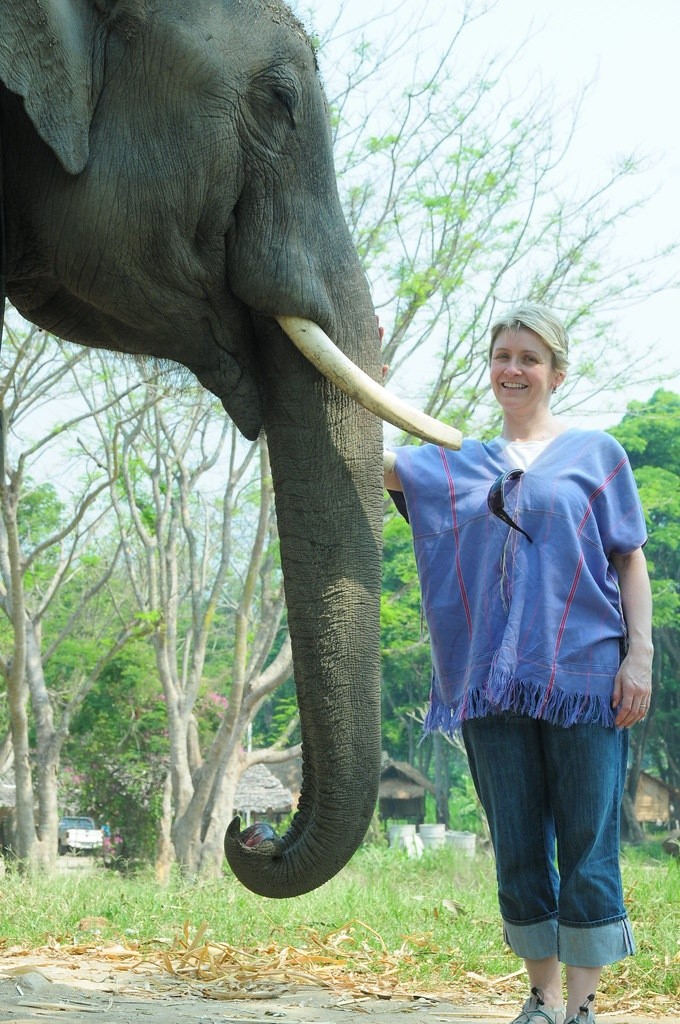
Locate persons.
[375,305,654,1024]
[100,821,124,854]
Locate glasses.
[486,468,533,545]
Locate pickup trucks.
[58,815,104,857]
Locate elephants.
[0,0,465,904]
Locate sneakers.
[561,994,597,1024]
[508,986,567,1024]
